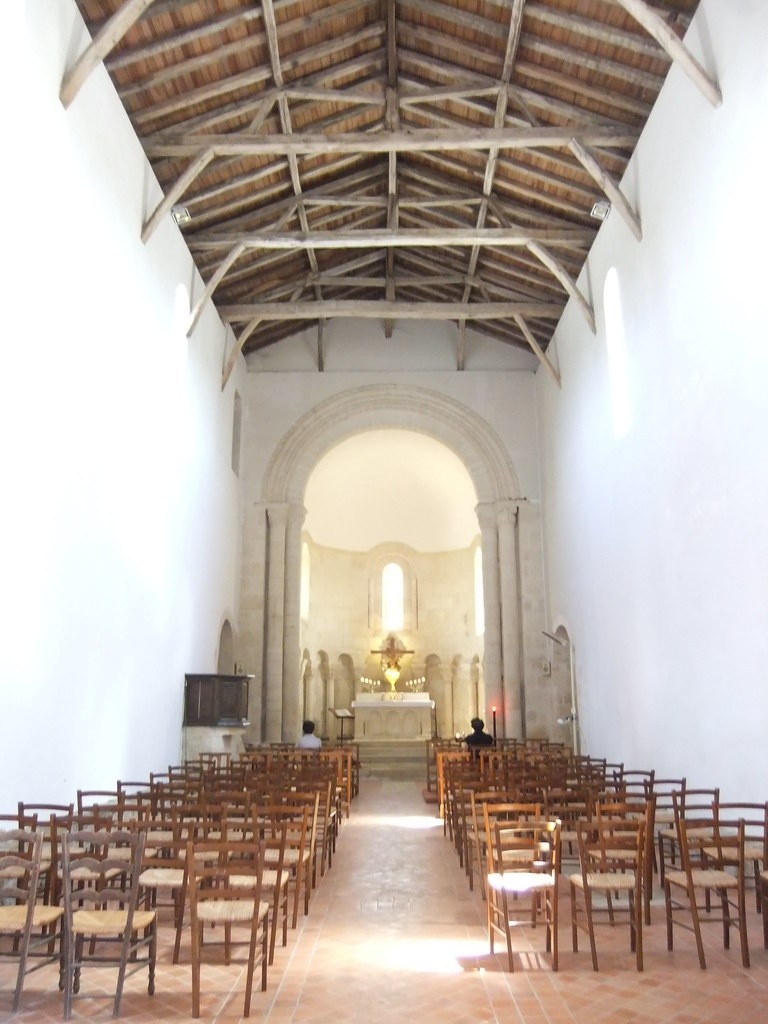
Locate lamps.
[590,200,612,221]
[171,205,192,225]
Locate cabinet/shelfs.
[182,673,255,727]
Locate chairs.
[0,740,365,1018]
[425,737,768,974]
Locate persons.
[295,720,322,749]
[464,718,494,761]
